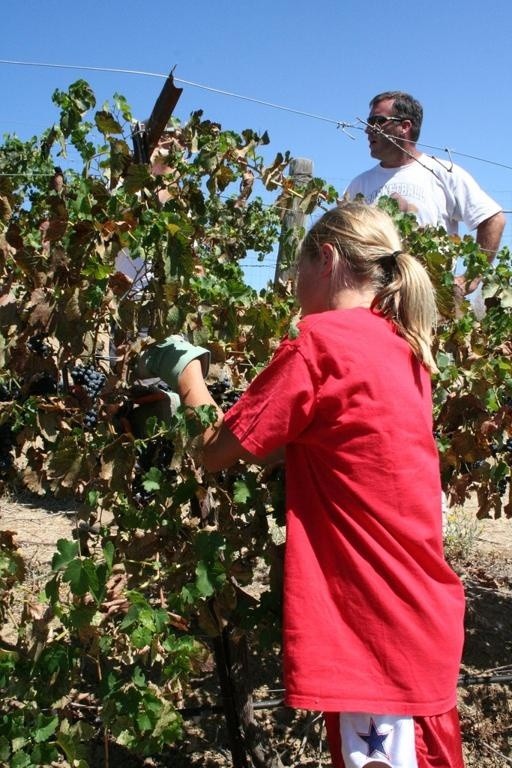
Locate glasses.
[367,115,401,125]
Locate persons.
[102,121,210,500]
[138,203,467,768]
[344,91,506,329]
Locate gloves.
[129,334,210,395]
